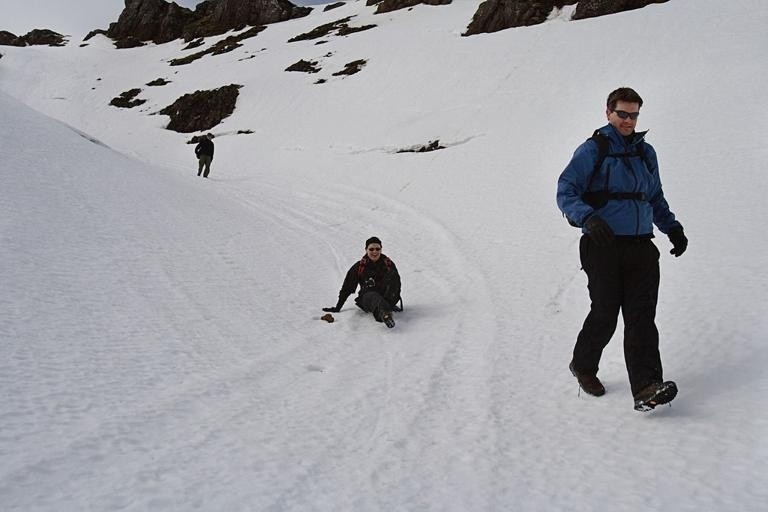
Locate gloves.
[670,235,688,257]
[322,307,340,313]
[586,215,616,248]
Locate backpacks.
[566,135,652,227]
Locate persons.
[195,133,215,179]
[557,88,688,413]
[322,237,401,330]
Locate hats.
[366,237,381,248]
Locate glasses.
[369,248,381,251]
[614,110,639,120]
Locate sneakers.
[570,360,605,396]
[634,381,677,411]
[383,314,395,328]
